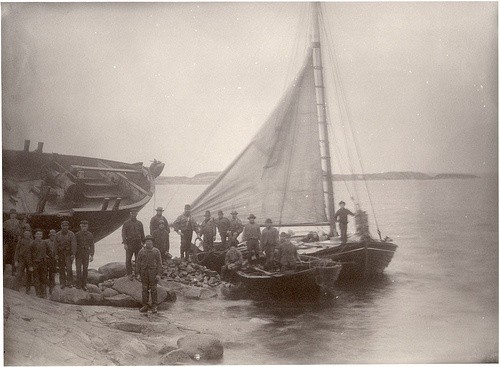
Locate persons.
[261,218,280,258]
[121,208,146,279]
[32,229,48,298]
[55,220,77,290]
[199,210,216,248]
[230,211,244,245]
[150,206,169,257]
[135,235,162,313]
[15,224,32,295]
[174,204,199,261]
[280,234,299,270]
[221,244,244,288]
[74,219,95,290]
[3,208,22,294]
[45,229,56,287]
[244,213,263,266]
[216,210,230,243]
[333,200,355,242]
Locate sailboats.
[171,2,398,280]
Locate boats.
[220,254,343,299]
[186,240,238,272]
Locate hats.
[155,206,164,211]
[158,219,165,225]
[185,204,191,211]
[231,210,237,214]
[143,234,155,241]
[218,211,223,215]
[280,232,285,236]
[129,208,138,211]
[48,229,56,236]
[284,234,291,238]
[265,218,272,222]
[247,214,256,220]
[339,201,345,204]
[204,210,210,216]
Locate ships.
[2,139,165,265]
